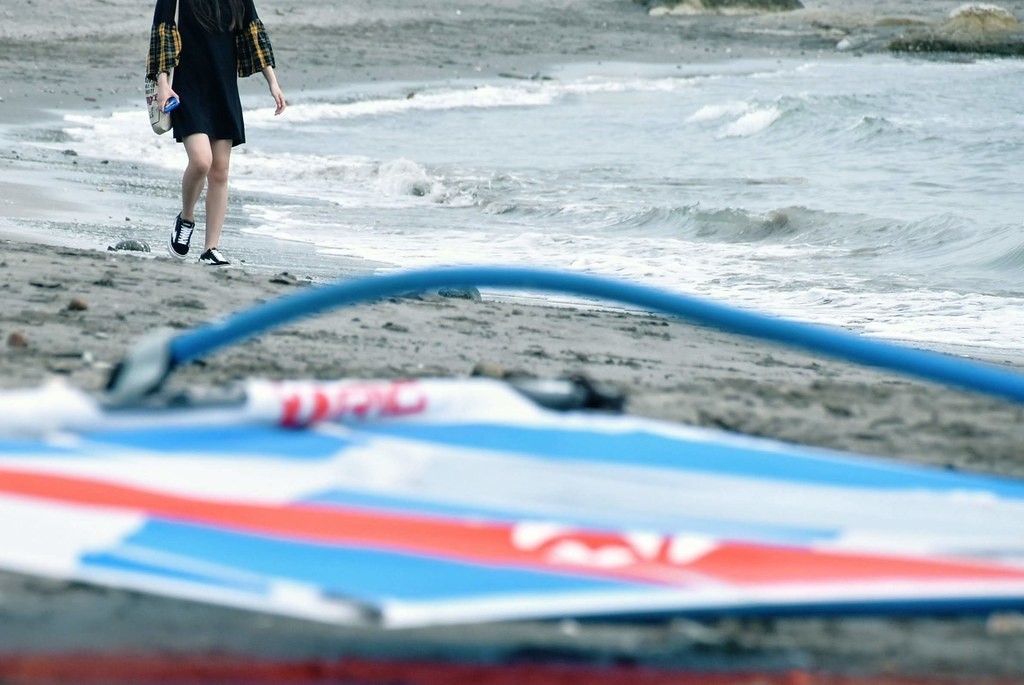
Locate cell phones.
[164,97,179,113]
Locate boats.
[1,261,1024,685]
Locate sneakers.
[198,247,230,268]
[168,211,196,260]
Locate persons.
[144,0,286,265]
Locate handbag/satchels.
[144,60,175,133]
